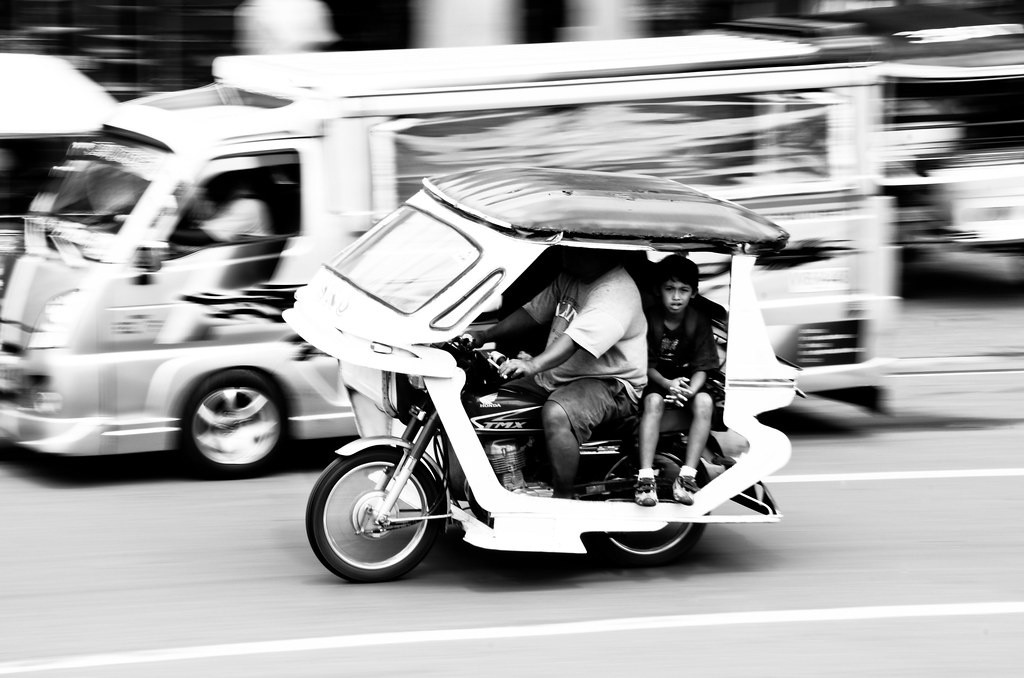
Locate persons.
[463,248,648,499]
[195,171,272,242]
[635,255,720,506]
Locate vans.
[885,24,1024,244]
[1,30,902,482]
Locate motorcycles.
[282,164,809,585]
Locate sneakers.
[672,476,698,505]
[633,475,658,506]
